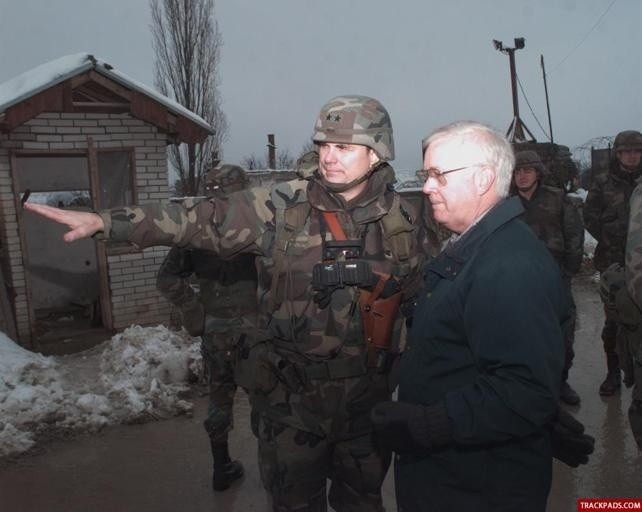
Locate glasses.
[415,166,480,185]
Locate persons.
[368,121,597,511]
[24,95,426,512]
[154,164,258,492]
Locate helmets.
[513,151,546,173]
[202,163,248,198]
[312,95,395,161]
[613,132,642,151]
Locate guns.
[359,273,398,366]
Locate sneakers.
[560,383,580,407]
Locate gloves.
[552,405,595,469]
[371,400,449,458]
[348,355,402,414]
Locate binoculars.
[312,240,371,290]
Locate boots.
[600,351,621,396]
[211,441,244,491]
